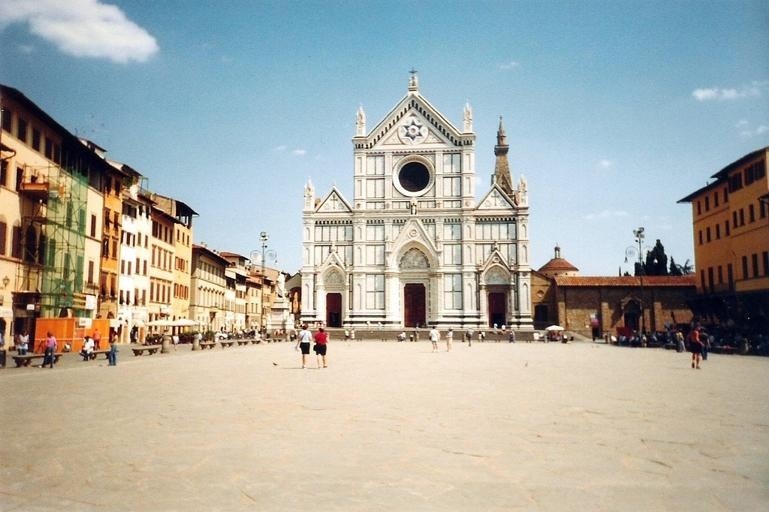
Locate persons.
[130,325,297,353]
[548,331,560,339]
[313,328,328,368]
[638,321,742,370]
[344,329,349,340]
[589,316,599,341]
[107,330,118,366]
[92,328,101,350]
[15,328,30,355]
[351,327,355,340]
[298,323,312,367]
[37,331,57,368]
[399,321,514,351]
[81,335,95,361]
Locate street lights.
[623,227,659,337]
[248,230,280,342]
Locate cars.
[145,330,296,347]
[612,322,769,356]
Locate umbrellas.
[546,325,565,331]
[144,318,212,334]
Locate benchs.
[78,348,116,360]
[12,352,62,368]
[132,346,160,358]
[190,326,283,350]
